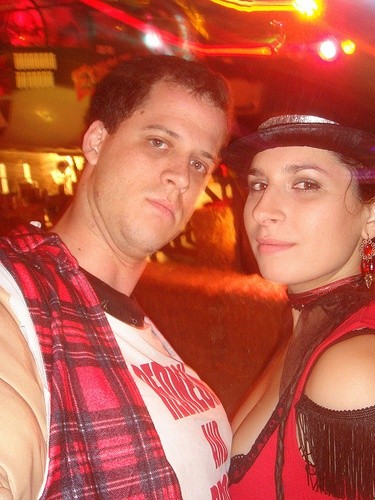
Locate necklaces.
[284,275,366,312]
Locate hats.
[219,81,375,172]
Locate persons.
[220,107,374,500]
[0,57,234,500]
